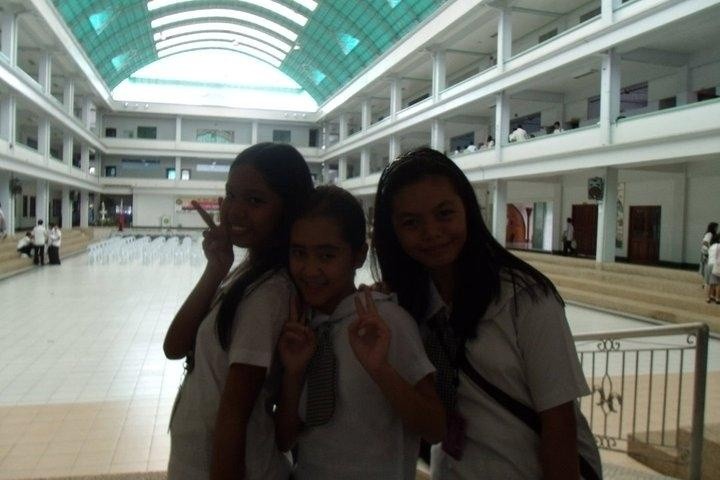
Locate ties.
[306,321,337,429]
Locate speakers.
[588,177,602,200]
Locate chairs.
[86,234,207,270]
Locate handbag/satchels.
[574,396,603,479]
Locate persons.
[563,217,574,256]
[369,143,604,480]
[163,142,314,480]
[272,184,450,480]
[450,136,497,153]
[507,120,562,141]
[17,219,62,265]
[701,222,720,304]
